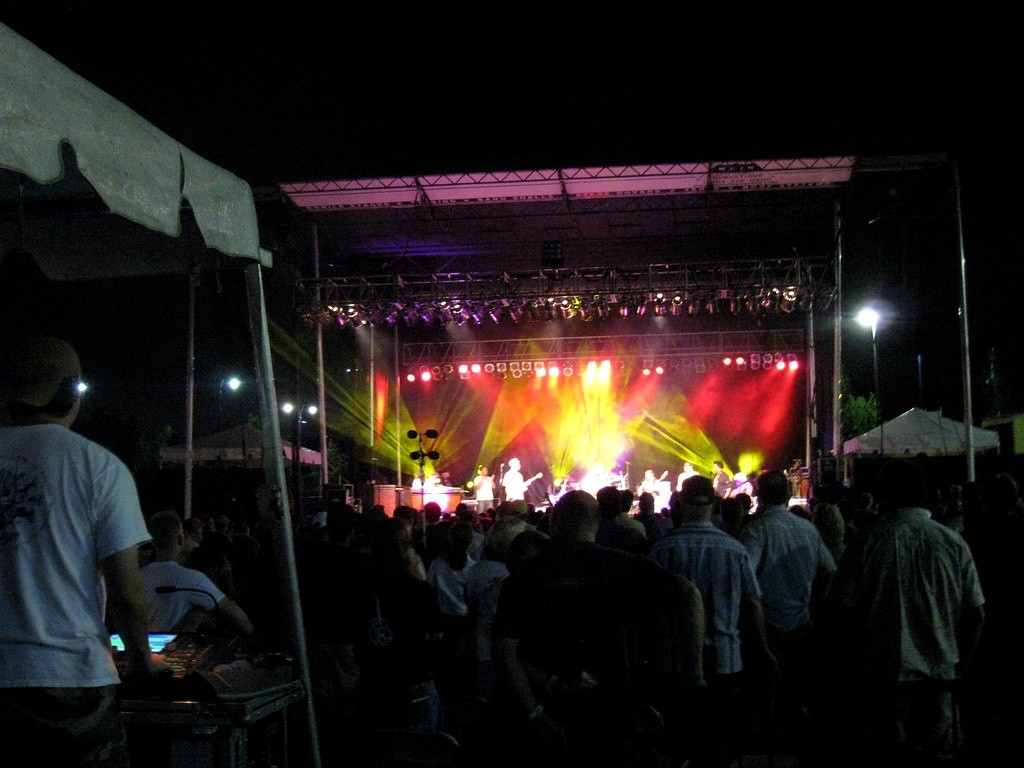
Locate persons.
[0,335,1024,768]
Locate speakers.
[369,485,395,518]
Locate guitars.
[645,470,668,493]
[505,472,544,497]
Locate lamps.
[563,360,584,376]
[781,287,797,313]
[484,363,507,380]
[763,354,773,370]
[418,365,453,382]
[818,283,839,311]
[459,371,470,380]
[316,299,470,327]
[643,359,667,375]
[750,354,761,370]
[509,287,779,321]
[670,358,705,373]
[774,352,797,363]
[510,361,532,379]
[534,361,544,370]
[469,303,486,325]
[487,301,506,324]
[798,287,816,309]
[547,361,557,369]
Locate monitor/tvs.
[109,633,178,653]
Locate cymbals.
[560,475,575,482]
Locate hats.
[184,518,204,533]
[5,335,81,407]
[682,476,715,507]
[597,486,634,519]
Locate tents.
[0,23,320,768]
[843,407,1000,490]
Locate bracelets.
[527,704,545,719]
